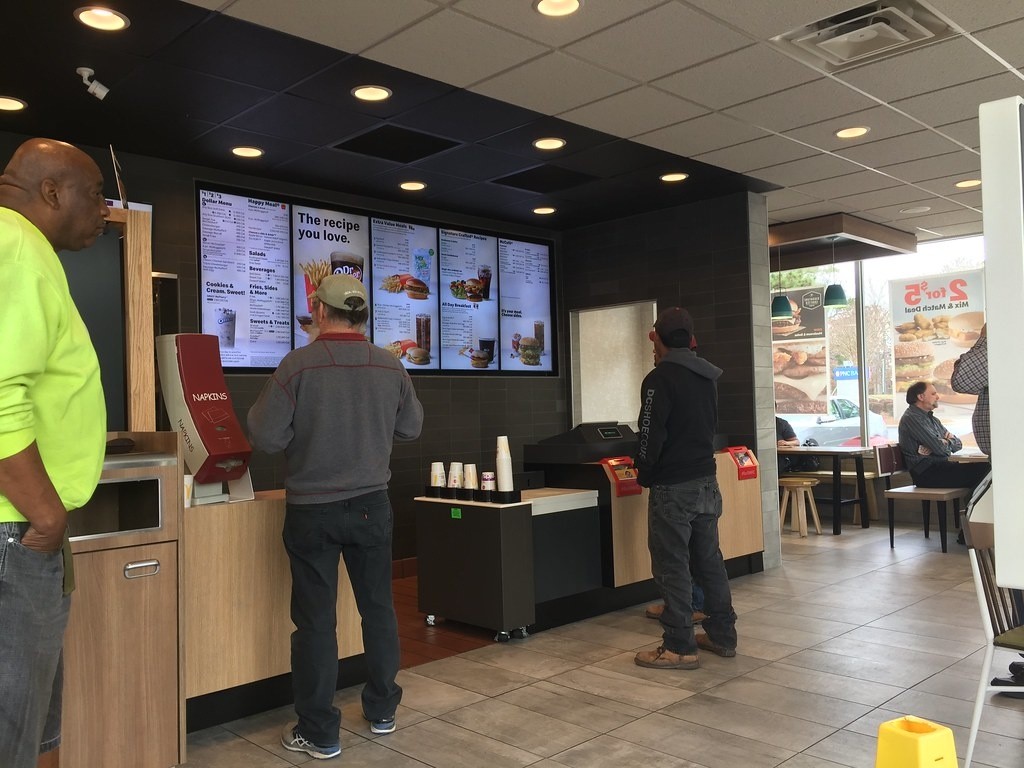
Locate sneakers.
[282,722,341,759]
[1009,661,1024,678]
[992,677,1024,699]
[692,611,708,624]
[695,632,737,656]
[634,646,699,669]
[646,604,664,618]
[363,705,396,733]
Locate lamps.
[823,236,847,307]
[772,246,793,321]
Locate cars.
[774,399,888,450]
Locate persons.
[774,400,799,446]
[897,381,985,546]
[0,136,112,768]
[950,318,1024,698]
[244,272,422,760]
[634,307,740,671]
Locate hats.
[307,275,369,311]
[653,307,694,341]
[650,328,698,349]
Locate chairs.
[958,510,1024,768]
[873,444,968,553]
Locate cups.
[534,321,544,356]
[478,336,499,364]
[412,247,431,293]
[464,464,479,489]
[215,309,236,348]
[330,252,364,283]
[183,475,195,508]
[430,461,446,487]
[481,472,495,491]
[477,265,492,300]
[447,462,464,488]
[496,436,514,491]
[416,313,431,353]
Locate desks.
[777,446,875,535]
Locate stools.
[778,477,822,537]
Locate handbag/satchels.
[797,439,821,470]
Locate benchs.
[779,470,879,525]
